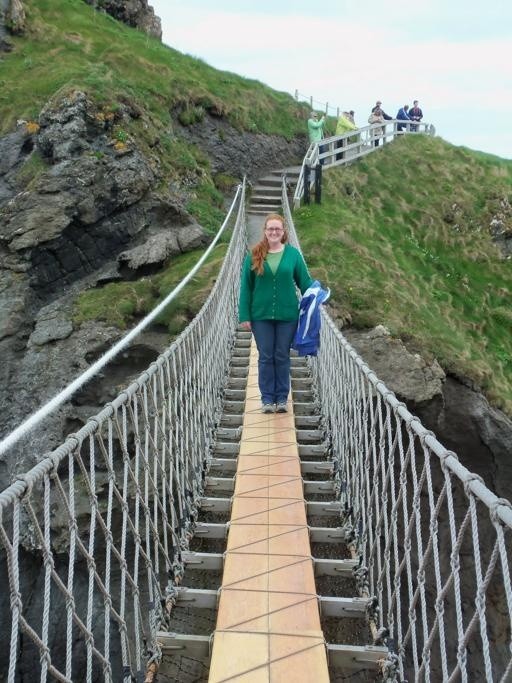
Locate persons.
[308,111,327,165]
[372,101,392,120]
[238,212,316,414]
[335,112,358,160]
[368,109,384,147]
[396,105,414,136]
[409,100,423,132]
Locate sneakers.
[276,403,288,413]
[262,403,275,414]
